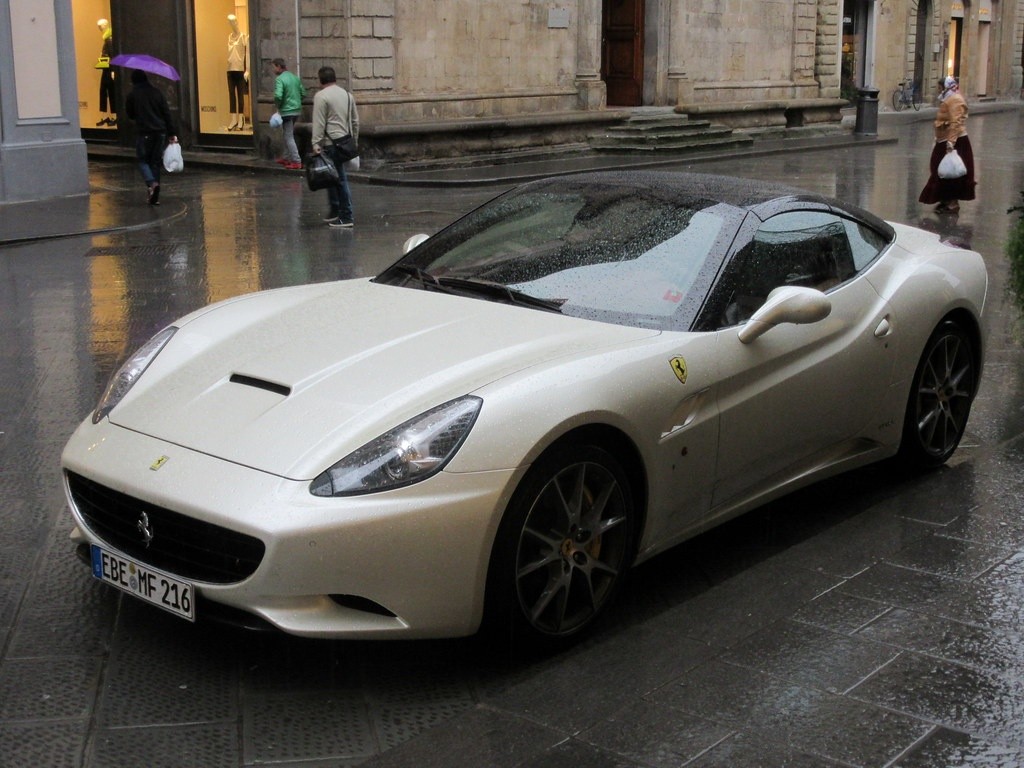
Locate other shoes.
[148,186,161,206]
[328,217,353,227]
[940,203,960,214]
[323,213,338,222]
[285,162,302,169]
[275,157,289,164]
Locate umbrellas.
[107,52,182,82]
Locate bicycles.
[892,78,922,112]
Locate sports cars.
[57,169,989,652]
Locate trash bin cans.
[854,84,880,137]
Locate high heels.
[237,117,245,131]
[108,118,116,126]
[96,118,110,126]
[228,121,238,131]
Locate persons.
[225,14,250,130]
[918,75,979,219]
[94,19,118,126]
[268,57,303,170]
[308,64,360,227]
[122,73,175,210]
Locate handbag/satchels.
[307,152,339,192]
[332,138,359,163]
[162,142,184,174]
[270,111,283,128]
[938,149,967,179]
[93,57,110,69]
